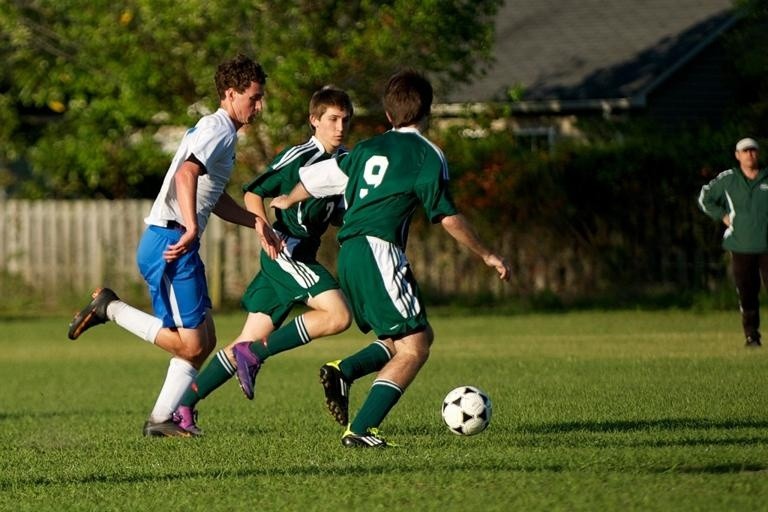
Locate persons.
[265,70,513,449]
[167,87,361,437]
[698,137,768,347]
[67,53,284,439]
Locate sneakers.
[143,420,195,440]
[231,342,262,401]
[319,359,351,426]
[340,428,410,450]
[67,286,120,341]
[171,404,206,437]
[744,335,762,349]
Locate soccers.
[441,386,492,436]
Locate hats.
[735,137,760,153]
